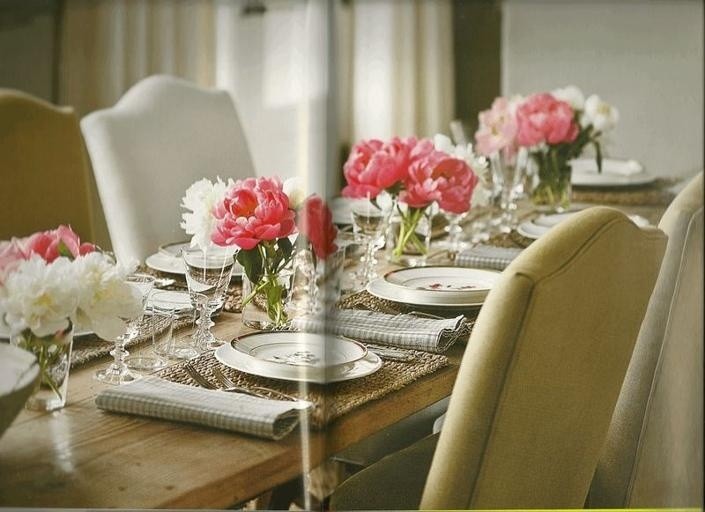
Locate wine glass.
[349,197,391,278]
[93,273,156,385]
[179,247,241,350]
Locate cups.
[332,231,368,305]
[151,292,208,359]
[392,201,433,254]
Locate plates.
[530,213,648,230]
[383,266,503,294]
[365,279,488,312]
[517,221,657,239]
[568,157,656,188]
[231,330,368,369]
[215,341,382,384]
[143,252,243,278]
[160,240,190,259]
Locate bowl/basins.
[0,340,40,435]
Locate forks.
[210,366,314,408]
[183,364,268,402]
